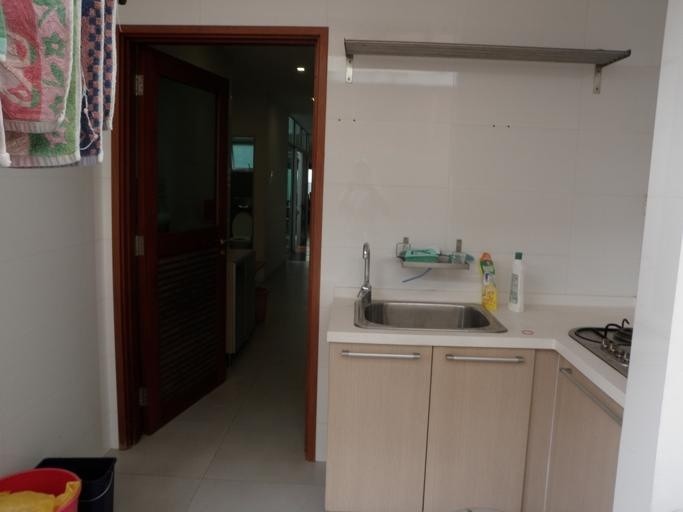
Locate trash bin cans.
[33,457,117,512]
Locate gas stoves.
[568,318,633,379]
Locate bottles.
[506,251,526,314]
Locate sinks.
[351,297,507,335]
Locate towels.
[0,0,81,168]
[81,0,116,163]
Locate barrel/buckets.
[33,454,117,512]
[0,469,82,512]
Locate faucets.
[361,242,372,303]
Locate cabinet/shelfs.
[535,349,625,512]
[226,249,255,365]
[325,343,536,512]
